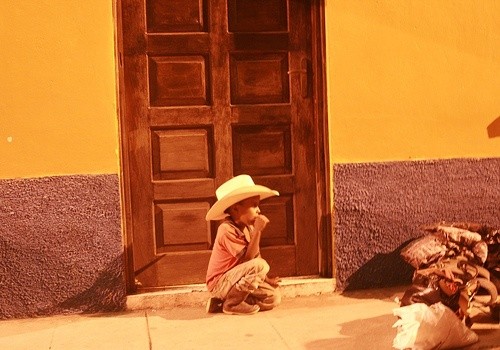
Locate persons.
[204,174,283,315]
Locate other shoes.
[208,298,223,312]
[222,301,260,314]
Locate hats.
[205,174,279,221]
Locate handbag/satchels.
[394,255,500,350]
